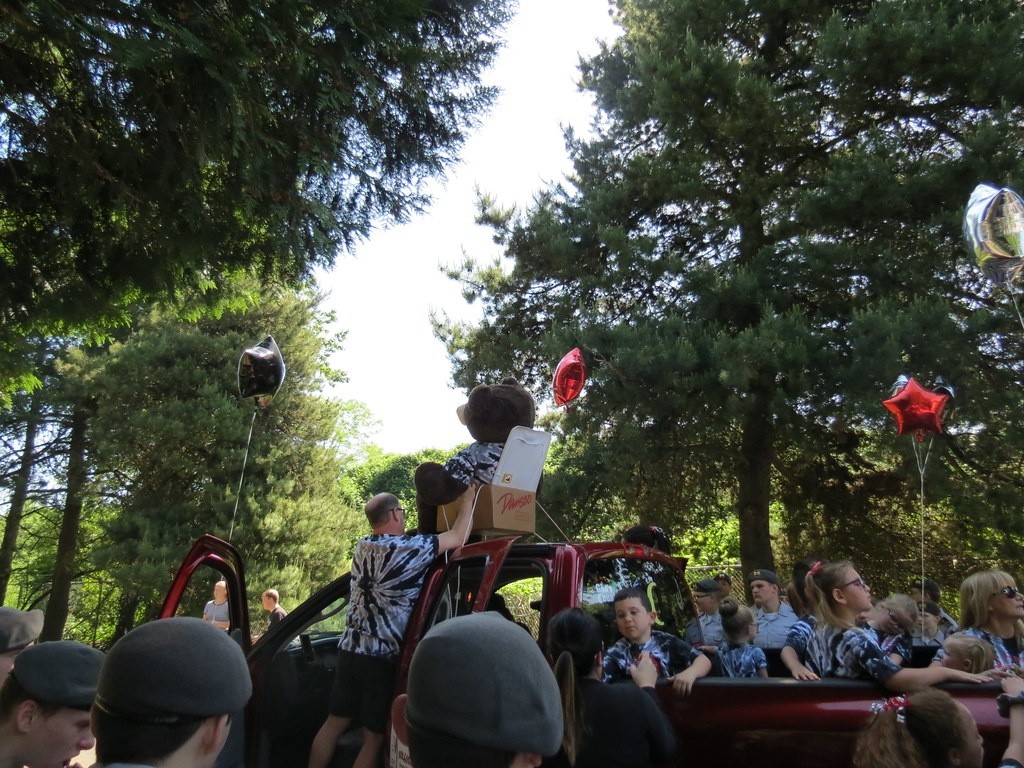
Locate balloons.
[553,348,587,413]
[962,182,1024,293]
[882,372,955,443]
[237,335,286,412]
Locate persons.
[0,641,107,768]
[308,483,476,768]
[392,611,563,768]
[780,559,1024,685]
[0,606,44,689]
[88,617,252,768]
[599,588,712,699]
[546,607,671,768]
[690,570,799,677]
[852,676,1024,768]
[250,588,287,646]
[203,581,230,630]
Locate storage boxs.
[437,486,536,534]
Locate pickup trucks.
[149,533,1010,768]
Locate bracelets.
[996,691,1024,719]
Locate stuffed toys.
[414,376,535,557]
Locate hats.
[14,640,107,709]
[0,606,45,652]
[749,569,781,590]
[406,611,564,758]
[910,578,941,592]
[94,616,253,721]
[690,579,720,592]
[712,573,732,586]
[917,601,941,615]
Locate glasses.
[693,594,712,600]
[888,608,907,634]
[389,508,406,518]
[993,586,1018,599]
[842,577,865,589]
